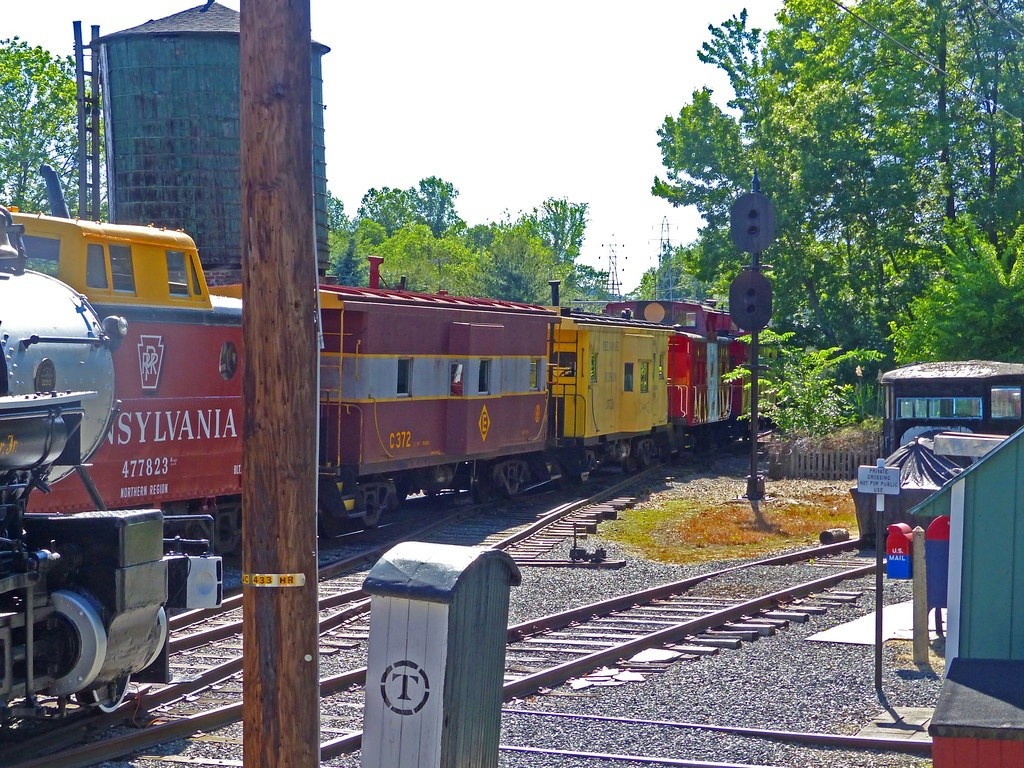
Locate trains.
[0,210,223,722]
[0,207,750,555]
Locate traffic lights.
[731,191,774,255]
[729,271,772,330]
